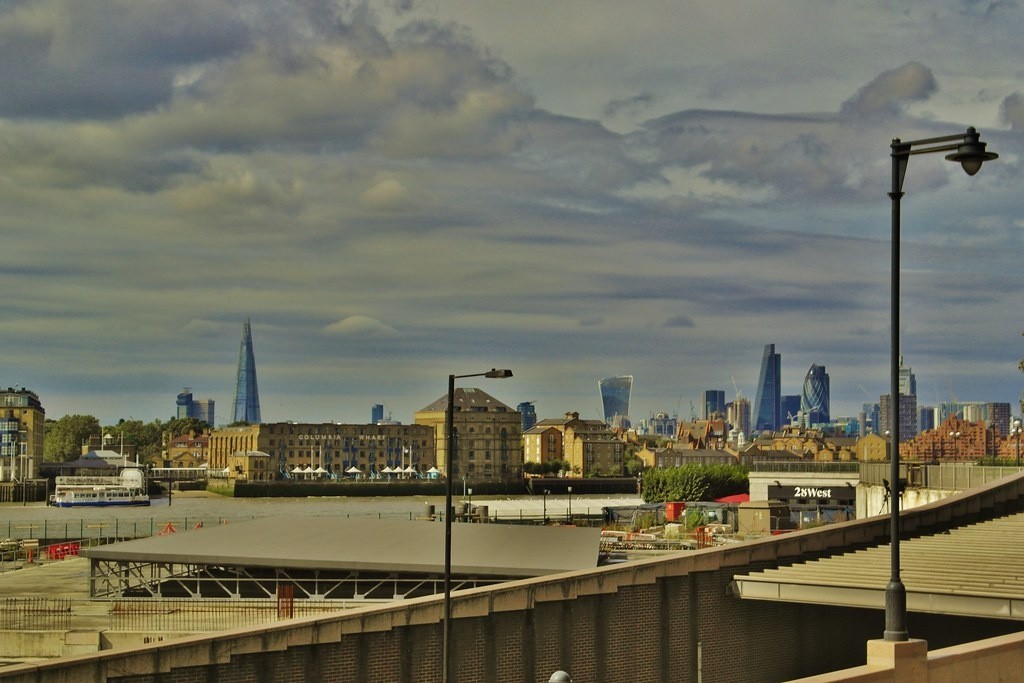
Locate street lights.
[1010,420,1022,466]
[864,126,1001,664]
[949,432,962,462]
[441,368,513,680]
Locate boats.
[49,483,151,508]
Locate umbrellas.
[302,467,316,473]
[380,466,393,473]
[314,467,327,473]
[290,467,303,473]
[392,466,403,472]
[404,466,417,472]
[426,467,439,473]
[346,466,362,473]
